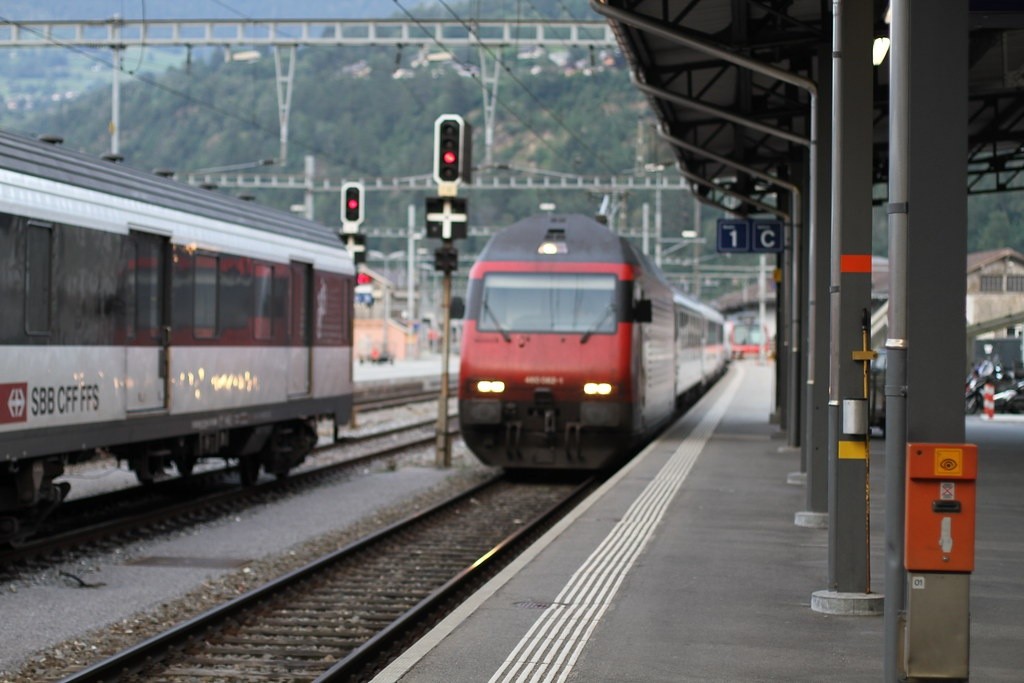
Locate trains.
[0,128,357,543]
[457,208,731,477]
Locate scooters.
[966,342,1024,418]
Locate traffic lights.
[447,248,458,271]
[433,113,465,187]
[341,181,365,226]
[434,247,446,272]
